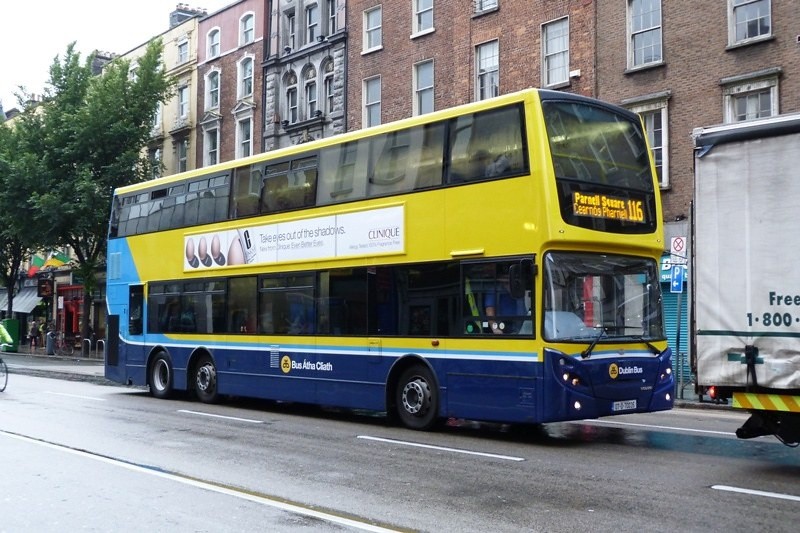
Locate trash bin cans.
[45,331,56,355]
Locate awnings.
[0,285,44,314]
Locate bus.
[100,82,680,433]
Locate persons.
[0,324,13,344]
[487,267,527,334]
[29,319,48,349]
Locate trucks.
[686,110,800,448]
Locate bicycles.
[0,341,14,393]
[49,331,74,356]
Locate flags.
[27,255,46,277]
[44,252,72,268]
